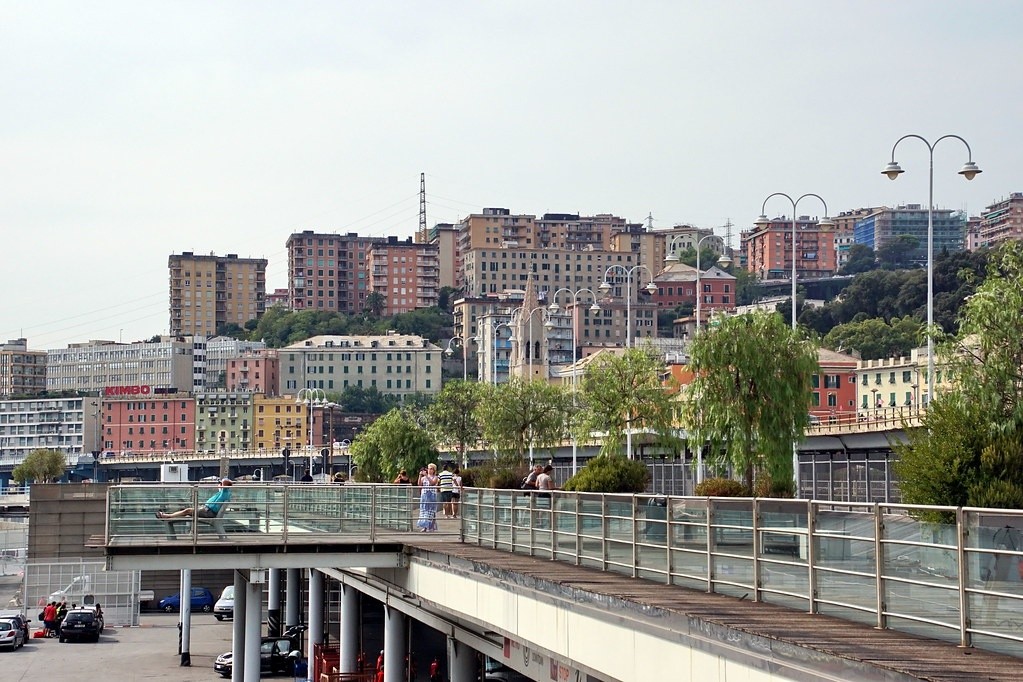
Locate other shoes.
[450,515,457,518]
[445,516,448,518]
[420,528,426,532]
[429,529,434,532]
[155,511,165,521]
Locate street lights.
[507,307,553,471]
[295,387,328,476]
[879,135,982,444]
[663,234,733,482]
[598,265,658,459]
[752,193,835,498]
[474,323,518,471]
[445,337,486,469]
[548,288,600,476]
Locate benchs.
[760,525,851,560]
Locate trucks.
[49,575,154,607]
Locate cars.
[59,609,103,643]
[158,587,214,613]
[213,626,308,677]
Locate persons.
[286,650,301,676]
[300,469,313,484]
[39,596,47,605]
[43,601,68,638]
[452,469,462,518]
[521,464,554,529]
[334,473,345,486]
[154,478,233,521]
[70,604,76,610]
[438,464,459,518]
[358,650,442,682]
[95,603,104,634]
[394,470,411,483]
[81,606,84,609]
[417,463,437,532]
[271,640,280,673]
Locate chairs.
[162,500,228,540]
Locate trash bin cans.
[646,498,673,541]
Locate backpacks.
[39,612,45,621]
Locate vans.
[214,586,234,621]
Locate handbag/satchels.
[33,632,44,638]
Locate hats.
[399,470,407,474]
[545,465,555,471]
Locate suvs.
[0,613,31,644]
[0,618,24,652]
